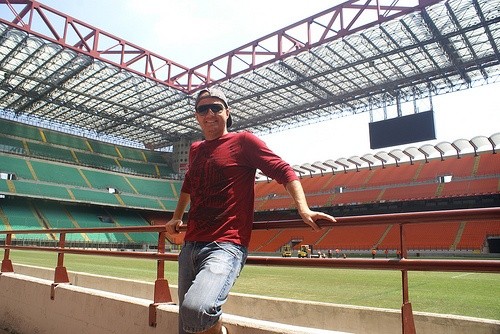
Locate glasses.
[196,104,225,113]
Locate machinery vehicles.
[298,245,309,258]
[281,245,291,257]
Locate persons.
[165,88,337,334]
[307,248,401,259]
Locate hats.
[195,88,232,128]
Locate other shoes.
[221,326,227,334]
